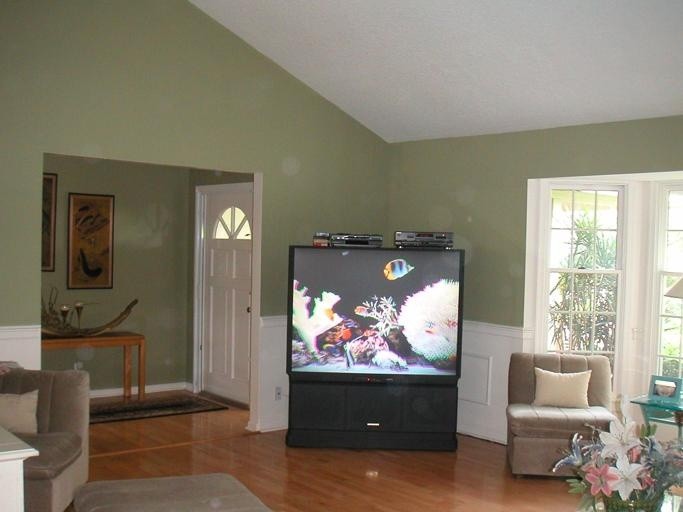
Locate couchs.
[1,361,92,511]
[506,353,618,481]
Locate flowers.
[551,417,683,497]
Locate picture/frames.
[68,192,113,289]
[42,171,58,271]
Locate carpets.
[90,395,226,424]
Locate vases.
[601,488,663,511]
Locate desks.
[0,425,40,512]
[41,331,146,402]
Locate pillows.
[533,366,592,409]
[1,390,39,435]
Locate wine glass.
[74,303,84,335]
[59,304,71,329]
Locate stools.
[76,472,273,512]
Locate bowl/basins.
[655,381,676,396]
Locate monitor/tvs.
[285,243,466,385]
